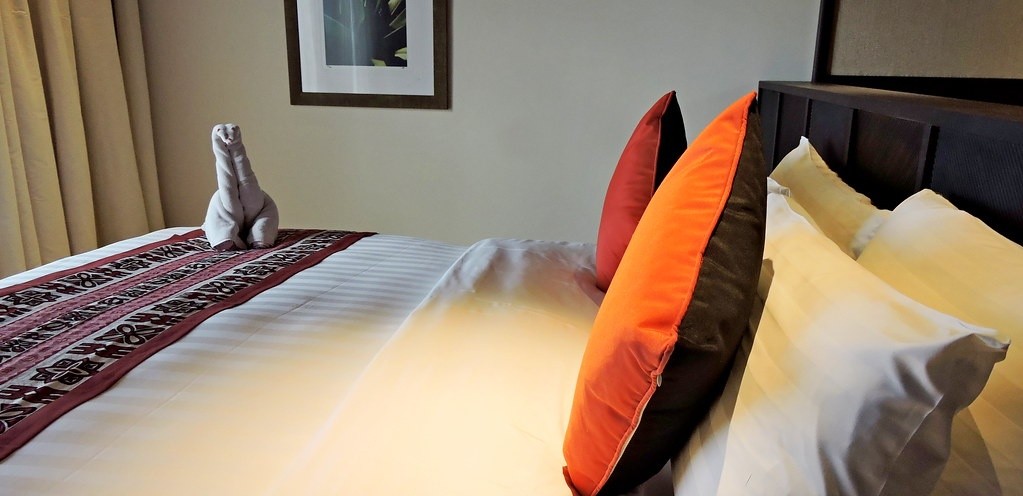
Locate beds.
[0,79,1023,496]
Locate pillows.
[595,90,689,295]
[669,174,1011,496]
[857,189,1023,496]
[769,135,892,259]
[562,90,766,496]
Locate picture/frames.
[284,0,450,109]
